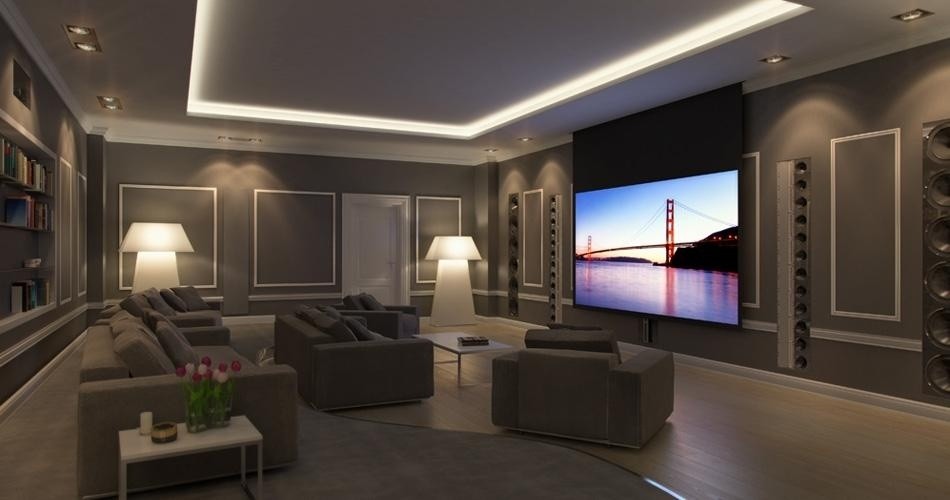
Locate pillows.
[112,286,202,370]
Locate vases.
[177,384,233,429]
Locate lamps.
[426,236,483,327]
[117,223,193,294]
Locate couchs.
[78,292,299,499]
[275,293,434,413]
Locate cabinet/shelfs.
[0,18,85,427]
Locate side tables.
[117,415,264,500]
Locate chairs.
[492,324,675,449]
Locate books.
[1,136,55,313]
[457,336,490,346]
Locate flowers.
[177,358,244,384]
